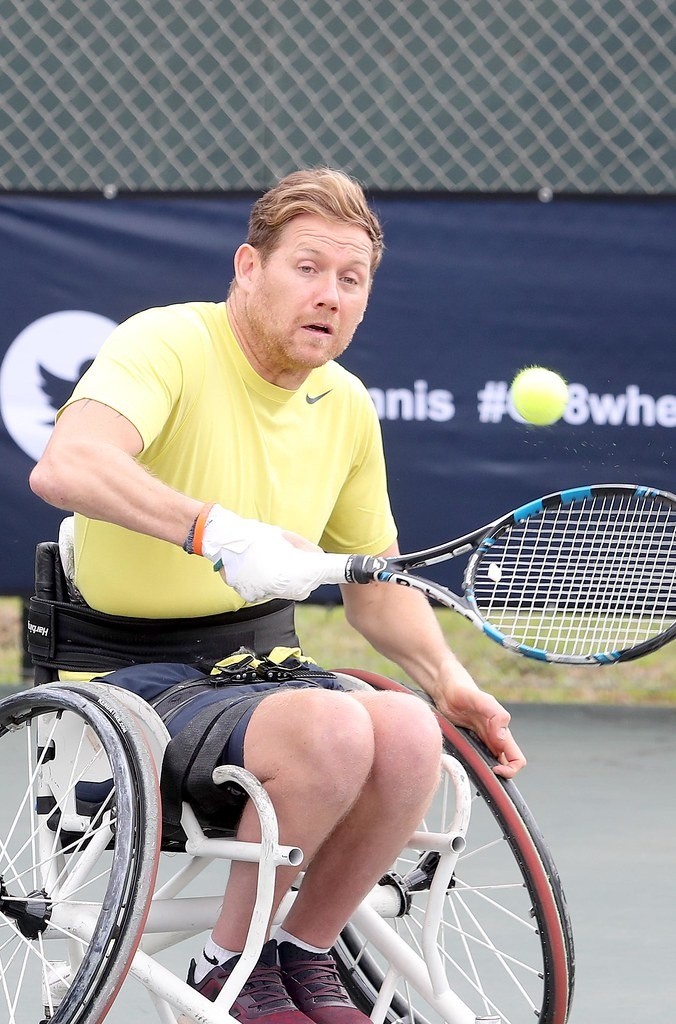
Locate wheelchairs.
[0,540,576,1024]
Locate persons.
[26,169,527,1024]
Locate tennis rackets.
[210,480,676,669]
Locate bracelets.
[193,501,217,557]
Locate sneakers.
[276,941,374,1024]
[186,939,315,1024]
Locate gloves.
[184,504,326,603]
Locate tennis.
[508,364,571,428]
